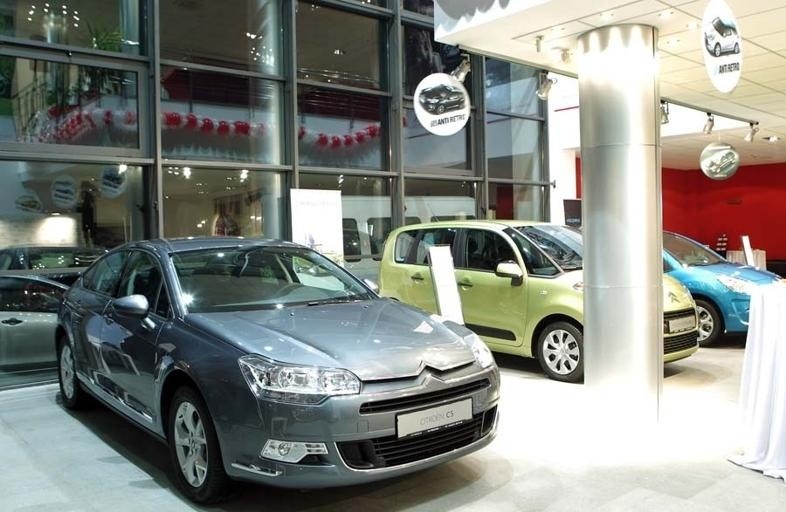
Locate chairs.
[234,250,292,284]
[499,236,534,274]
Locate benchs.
[137,257,238,310]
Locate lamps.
[450,52,471,84]
[535,73,558,101]
[660,101,670,124]
[536,35,544,52]
[702,115,715,135]
[744,123,760,144]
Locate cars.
[0,268,73,377]
[661,225,785,348]
[704,149,734,173]
[0,241,116,286]
[51,234,504,507]
[375,217,705,389]
[706,16,741,54]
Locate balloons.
[22,97,411,150]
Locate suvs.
[416,83,468,118]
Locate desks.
[726,249,767,271]
[727,278,786,480]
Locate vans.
[341,195,475,292]
